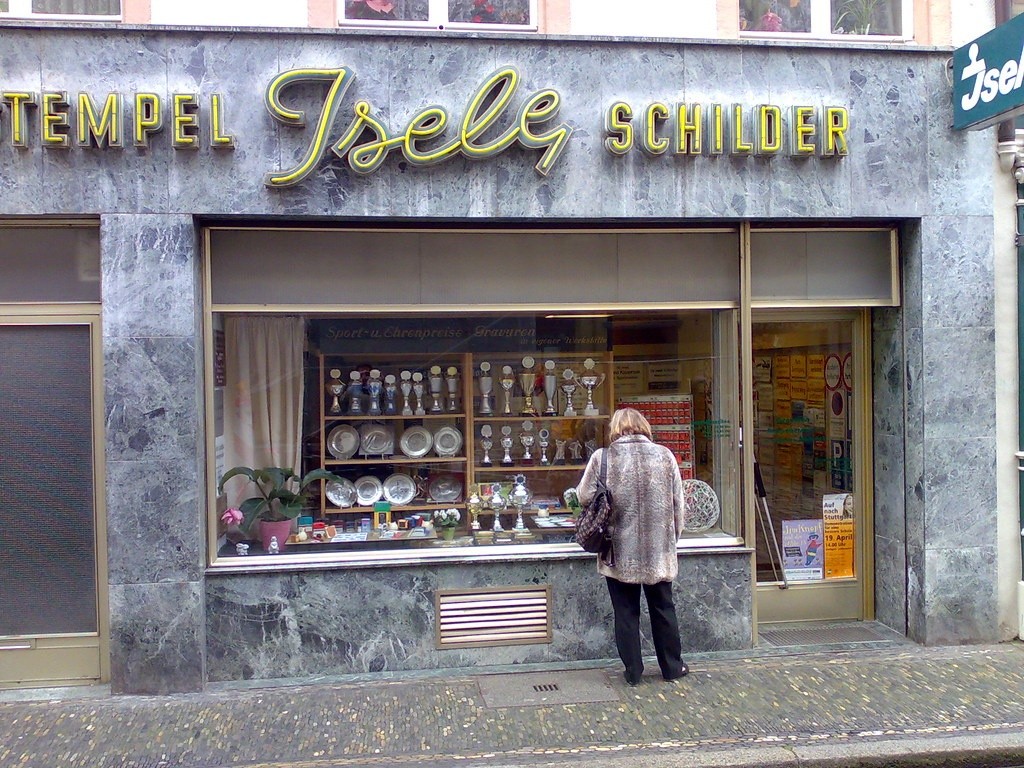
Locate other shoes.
[625,671,641,686]
[664,664,689,679]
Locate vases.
[573,505,584,519]
[258,517,292,551]
[441,525,454,542]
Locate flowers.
[218,462,335,527]
[564,488,580,507]
[434,507,460,525]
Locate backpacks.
[574,481,621,567]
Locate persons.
[576,408,690,686]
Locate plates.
[433,425,463,456]
[325,477,358,507]
[399,425,432,457]
[327,424,360,458]
[361,426,393,454]
[429,476,462,500]
[353,476,383,506]
[382,473,417,506]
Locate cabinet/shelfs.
[318,351,468,516]
[472,349,617,496]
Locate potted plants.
[834,0,886,35]
